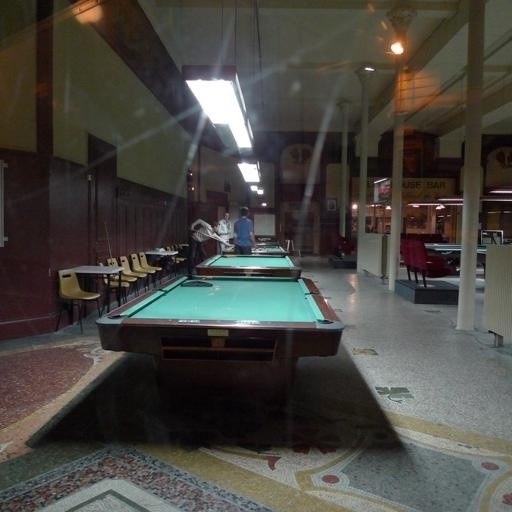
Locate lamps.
[183,0,264,195]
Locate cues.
[195,232,236,247]
[103,220,116,282]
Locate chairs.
[54,269,101,335]
[98,243,189,318]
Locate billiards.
[251,246,267,252]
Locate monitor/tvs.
[373,177,392,204]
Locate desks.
[194,242,303,277]
[70,265,124,325]
[423,242,512,280]
[95,272,346,410]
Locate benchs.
[400,238,458,288]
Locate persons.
[233,207,257,255]
[187,218,232,272]
[217,212,234,255]
